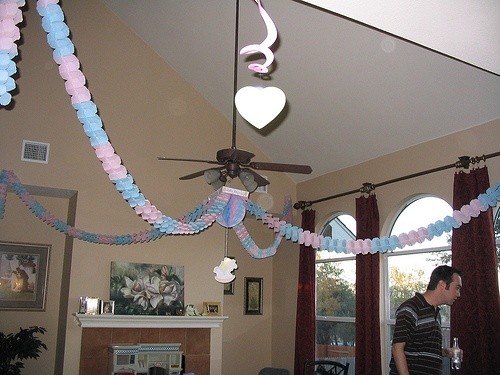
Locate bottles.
[451,336,461,369]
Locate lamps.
[204,163,258,192]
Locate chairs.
[303,360,349,375]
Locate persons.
[389,265,462,375]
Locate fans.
[155,2,311,187]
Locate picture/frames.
[203,302,221,316]
[0,242,52,312]
[224,256,234,295]
[243,277,263,315]
[101,300,115,315]
